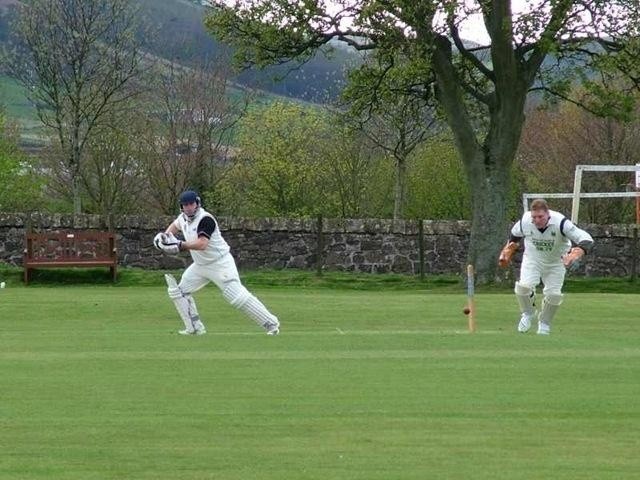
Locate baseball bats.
[467,265,474,332]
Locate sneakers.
[267,321,280,335]
[537,320,550,334]
[518,310,536,332]
[179,324,206,334]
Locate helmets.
[178,192,196,206]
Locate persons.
[498,199,594,335]
[151,190,281,336]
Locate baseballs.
[463,308,470,314]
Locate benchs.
[24,229,117,283]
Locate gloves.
[154,232,183,254]
